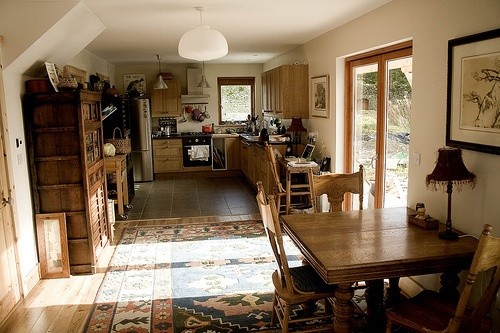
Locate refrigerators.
[123,98,154,182]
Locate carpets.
[82,219,410,333]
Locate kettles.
[260,128,270,145]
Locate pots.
[202,125,212,132]
[185,105,211,122]
[158,125,172,136]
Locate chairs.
[256,141,500,333]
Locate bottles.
[415,202,426,219]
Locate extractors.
[180,69,210,104]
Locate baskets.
[105,127,131,154]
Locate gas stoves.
[181,131,211,137]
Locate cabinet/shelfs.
[149,79,182,117]
[152,138,304,198]
[261,64,310,119]
[21,87,111,274]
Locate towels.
[190,144,210,161]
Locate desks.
[278,206,480,333]
[103,153,133,220]
[276,156,320,215]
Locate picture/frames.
[301,144,315,160]
[446,27,500,155]
[311,74,330,118]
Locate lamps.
[177,6,228,61]
[153,53,168,90]
[425,147,478,240]
[286,117,307,158]
[197,61,211,88]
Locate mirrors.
[35,212,70,279]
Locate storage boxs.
[408,213,441,230]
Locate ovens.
[182,135,211,166]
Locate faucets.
[112,126,122,139]
[257,119,269,130]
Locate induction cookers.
[156,131,181,140]
